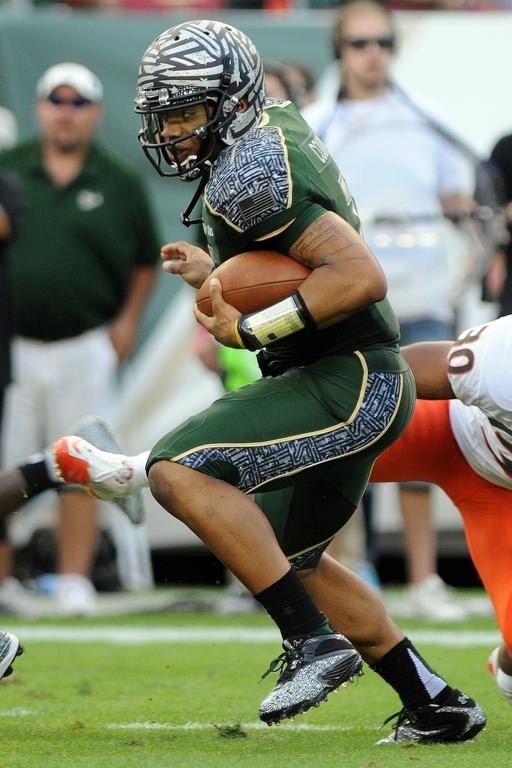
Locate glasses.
[48,94,90,106]
[344,34,394,49]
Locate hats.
[36,64,106,106]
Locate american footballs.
[197,251,311,316]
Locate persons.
[133,17,488,752]
[1,416,145,683]
[3,0,511,624]
[40,309,511,700]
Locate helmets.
[133,19,265,181]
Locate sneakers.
[55,418,142,523]
[1,630,23,681]
[375,686,487,745]
[487,645,512,697]
[258,630,364,727]
